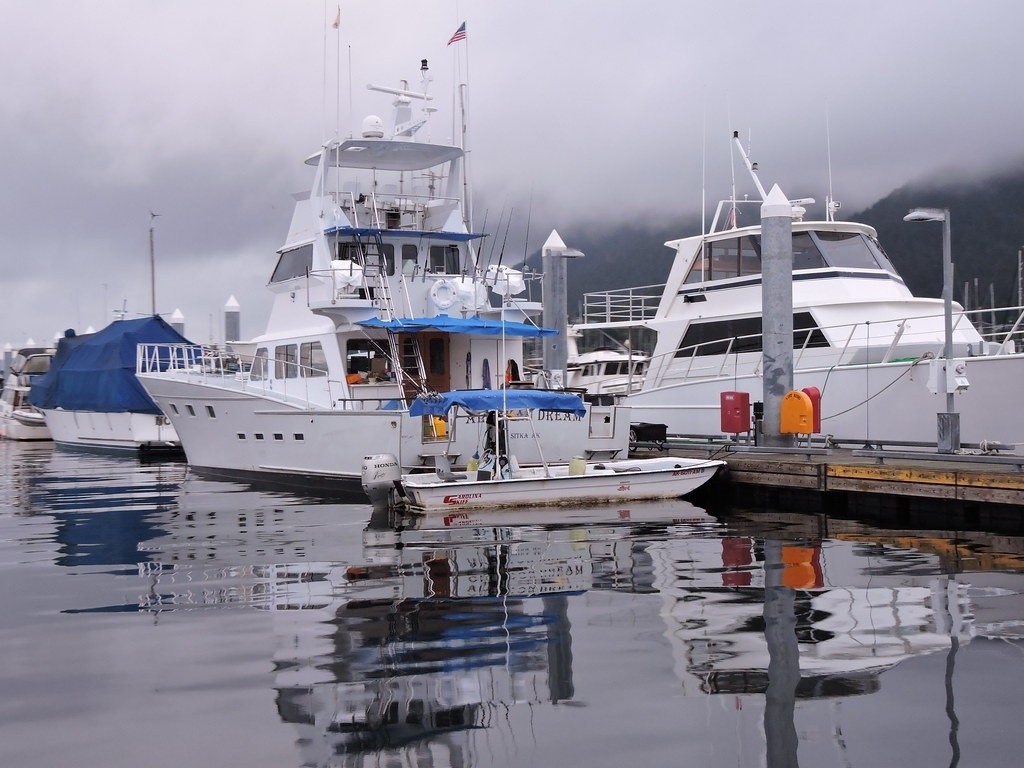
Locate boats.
[413,500,722,533]
[23,210,221,450]
[527,97,1024,451]
[0,352,64,441]
[132,6,557,479]
[395,271,731,515]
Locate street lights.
[903,202,962,450]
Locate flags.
[332,8,340,29]
[447,22,467,47]
